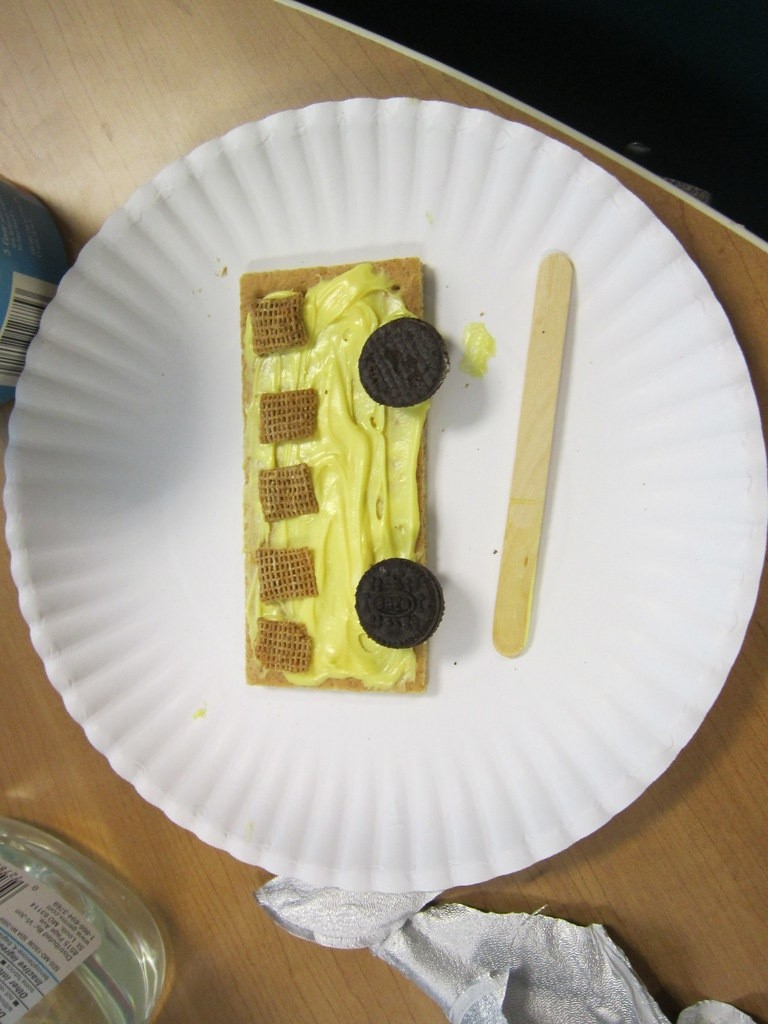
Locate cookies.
[239,257,428,691]
[355,557,444,649]
[360,319,449,408]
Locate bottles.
[1,817,165,1024]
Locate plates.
[4,97,768,894]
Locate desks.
[0,0,768,1024]
